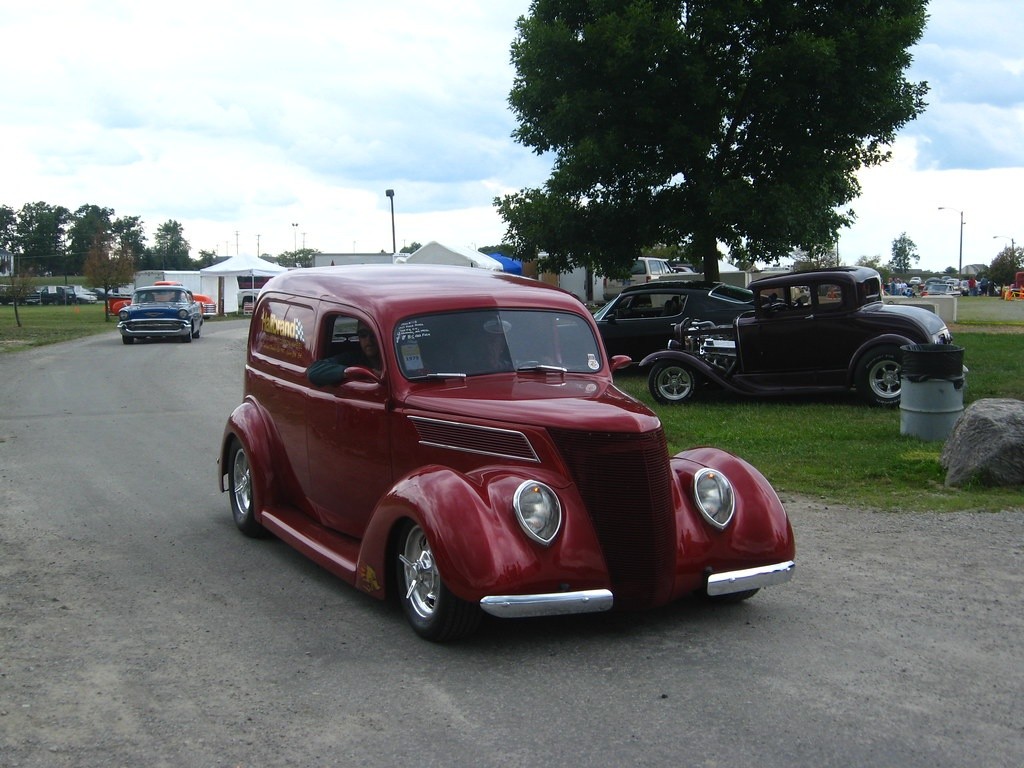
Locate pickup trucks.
[109,280,218,319]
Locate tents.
[199,254,289,308]
[402,240,522,274]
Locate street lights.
[292,222,298,254]
[993,236,1016,275]
[938,206,963,281]
[385,189,396,252]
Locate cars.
[924,275,998,297]
[909,277,922,285]
[117,285,204,344]
[91,287,112,301]
[556,277,796,364]
[217,264,795,645]
[639,265,969,410]
[0,282,97,306]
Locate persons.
[891,277,911,296]
[448,333,513,376]
[306,319,383,387]
[968,275,995,296]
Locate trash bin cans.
[899,343,964,444]
